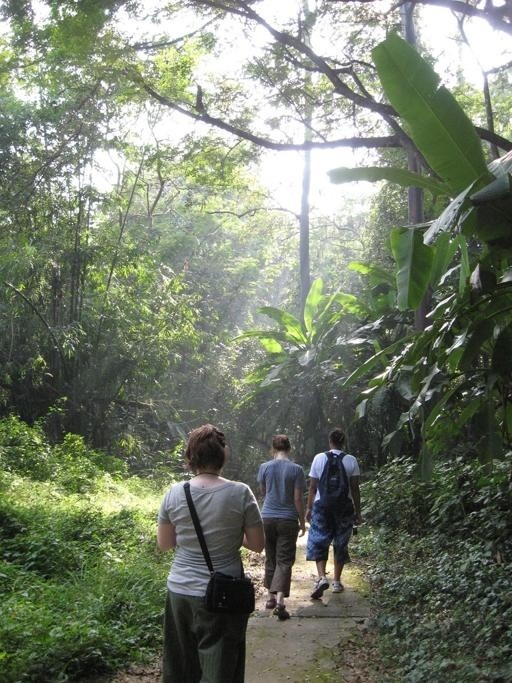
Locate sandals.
[274,604,289,619]
[265,598,276,608]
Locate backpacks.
[318,452,354,513]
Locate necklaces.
[196,472,218,476]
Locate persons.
[306,427,363,599]
[156,424,267,683]
[257,434,307,620]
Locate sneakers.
[332,582,344,593]
[310,576,329,598]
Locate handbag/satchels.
[205,571,256,614]
